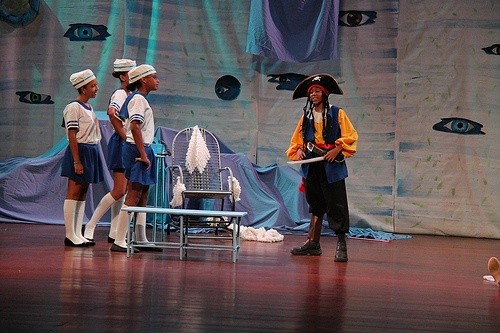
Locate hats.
[113,59,136,72]
[70,69,96,89]
[128,64,157,83]
[293,73,344,99]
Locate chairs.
[164,124,242,259]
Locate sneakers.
[335,241,347,261]
[290,240,322,256]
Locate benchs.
[118,206,249,262]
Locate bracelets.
[74,161,81,164]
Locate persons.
[60,69,104,247]
[82,58,137,243]
[110,65,163,252]
[286,73,358,262]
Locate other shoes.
[111,244,140,253]
[64,237,87,246]
[107,236,133,243]
[82,226,93,241]
[137,246,163,251]
[86,241,96,246]
